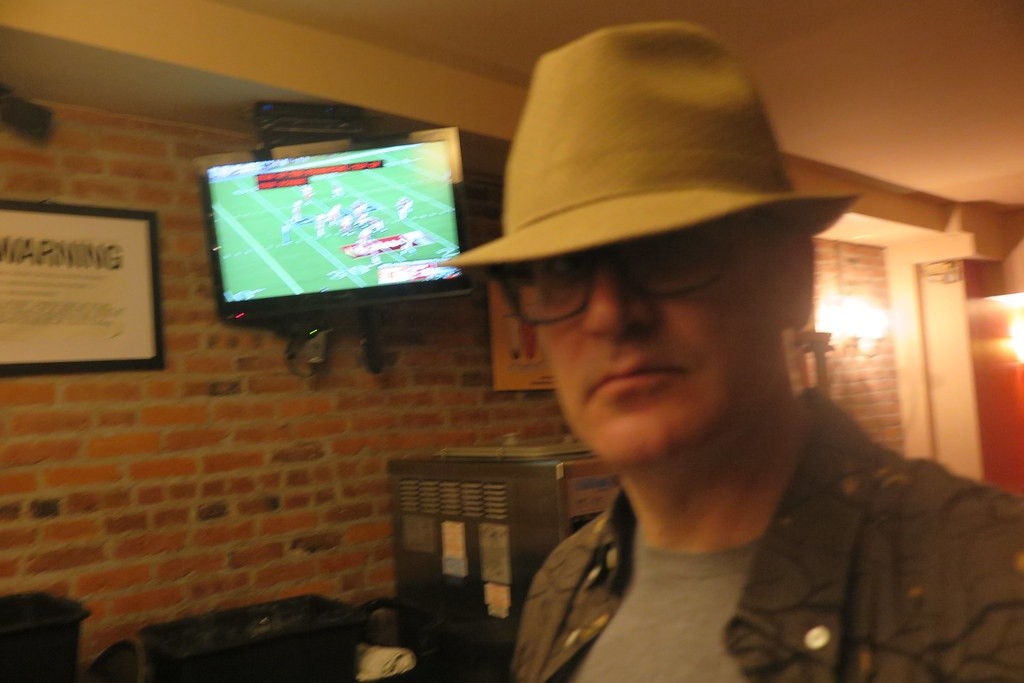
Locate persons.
[436,25,1024,682]
[282,185,417,264]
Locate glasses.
[495,222,801,326]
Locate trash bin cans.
[137,593,369,683]
[0,590,92,683]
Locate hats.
[437,19,860,279]
